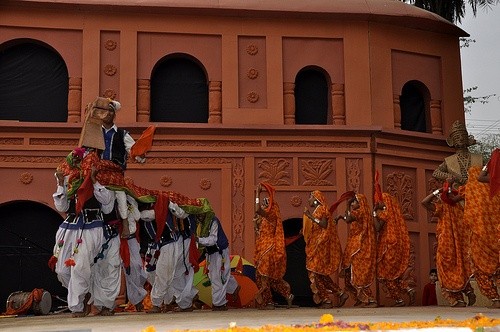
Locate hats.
[109,101,121,111]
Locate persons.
[253,182,294,310]
[432,119,475,186]
[373,193,415,307]
[303,191,349,308]
[84,98,146,238]
[335,193,378,308]
[53,164,240,318]
[422,149,500,309]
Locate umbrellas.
[193,255,259,309]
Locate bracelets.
[315,219,320,223]
[482,166,488,171]
[256,198,259,203]
[373,212,376,217]
[433,190,440,195]
